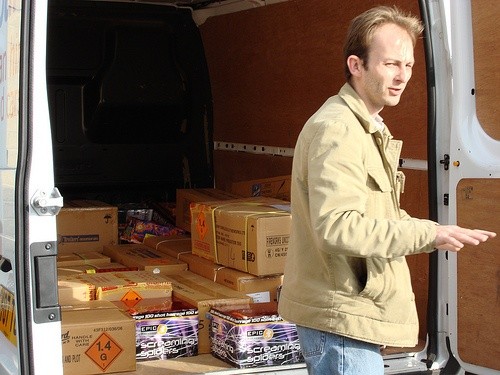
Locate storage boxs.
[58,175,306,375]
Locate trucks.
[1,0,500,375]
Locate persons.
[277,7,496,374]
